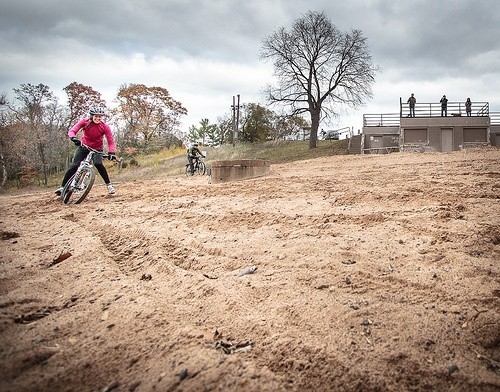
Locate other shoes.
[195,166,199,168]
[191,169,196,173]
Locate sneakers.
[107,183,116,194]
[55,186,64,196]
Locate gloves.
[71,137,81,146]
[108,152,116,160]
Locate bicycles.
[57,142,120,207]
[186,156,206,176]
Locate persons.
[465,98,472,116]
[188,144,205,173]
[55,107,116,196]
[407,94,416,117]
[320,129,326,139]
[440,95,448,116]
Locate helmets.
[89,106,105,115]
